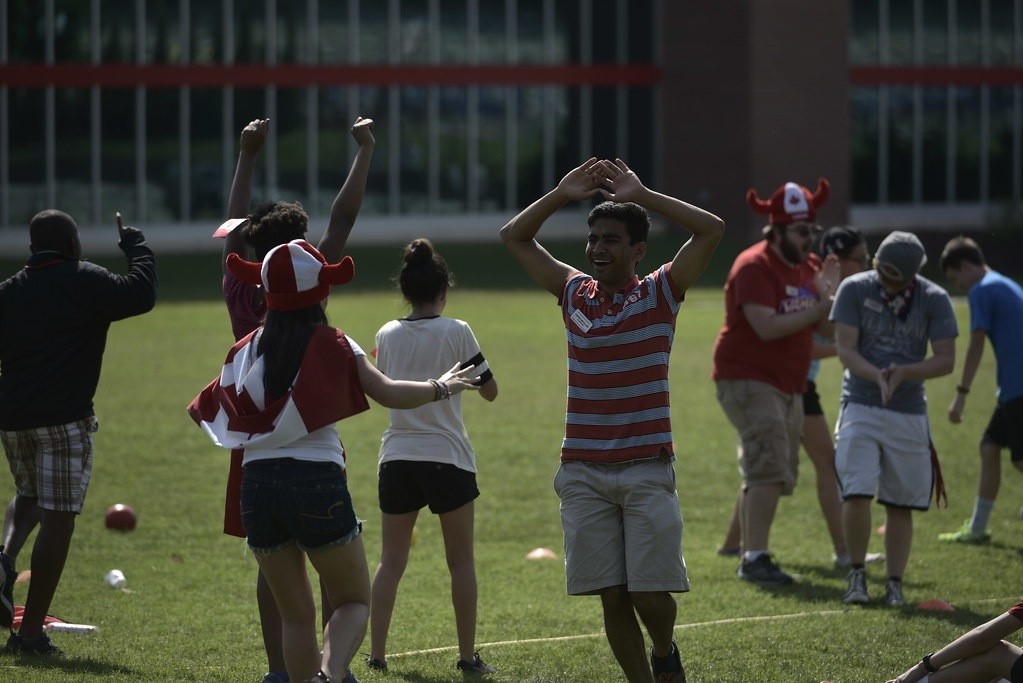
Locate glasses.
[847,253,870,266]
[789,226,822,239]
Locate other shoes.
[833,548,885,566]
[262,672,291,683]
[717,543,741,556]
[310,668,360,683]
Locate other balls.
[106,504,136,531]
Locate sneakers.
[842,568,869,605]
[6,630,64,656]
[940,520,991,547]
[0,548,18,629]
[458,647,496,675]
[884,582,906,610]
[650,640,688,683]
[366,658,389,671]
[739,552,796,588]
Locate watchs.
[923,653,939,672]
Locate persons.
[0,209,156,655]
[499,157,724,683]
[221,117,376,683]
[186,239,481,683]
[711,179,1023,683]
[364,240,498,675]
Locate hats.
[224,243,353,309]
[873,230,927,281]
[748,174,829,222]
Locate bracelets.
[428,379,451,401]
[956,386,970,394]
